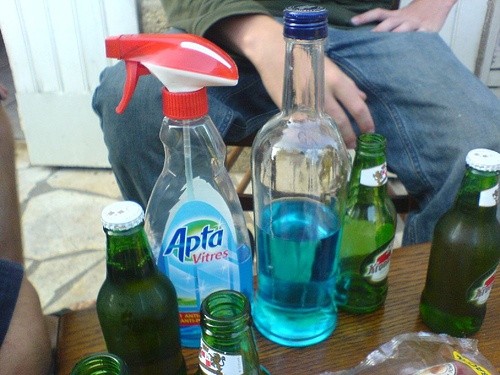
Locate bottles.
[194,289,272,374]
[333,133,396,316]
[247,2,351,349]
[416,147,500,339]
[71,353,134,374]
[95,199,189,375]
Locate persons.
[91,1,499,247]
[0,258,54,375]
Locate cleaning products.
[102,33,268,351]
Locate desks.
[40,239,500,373]
